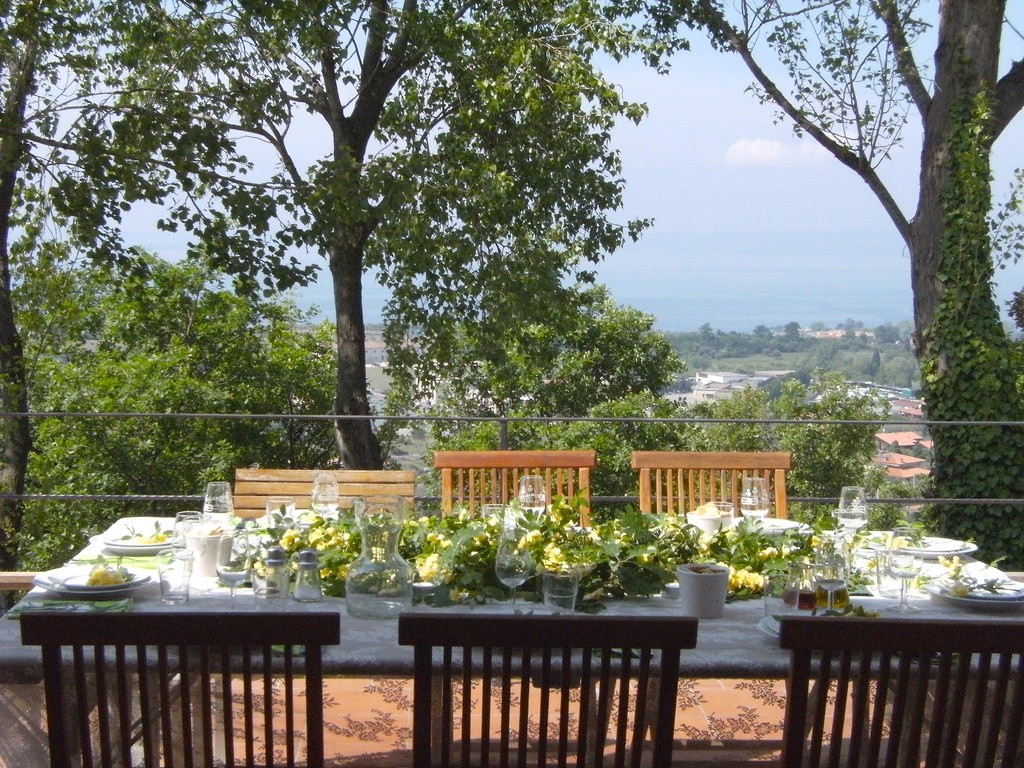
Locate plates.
[835,531,979,560]
[923,578,1024,609]
[264,509,341,529]
[758,612,881,639]
[33,566,152,598]
[103,530,182,556]
[732,517,809,536]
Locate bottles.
[293,548,322,602]
[265,547,285,598]
[346,493,413,620]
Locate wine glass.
[495,530,531,614]
[838,486,868,542]
[813,535,850,617]
[171,511,206,595]
[215,529,250,610]
[887,527,924,615]
[310,472,339,526]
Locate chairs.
[778,615,1023,768]
[19,605,341,767]
[234,469,414,518]
[397,614,698,768]
[632,450,793,520]
[433,448,597,523]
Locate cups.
[186,528,235,578]
[783,559,850,610]
[741,477,770,522]
[764,574,801,627]
[266,498,295,529]
[482,504,513,537]
[519,474,546,522]
[707,501,734,531]
[157,549,194,606]
[686,511,721,543]
[542,569,578,615]
[676,564,730,618]
[877,550,914,598]
[202,482,234,528]
[252,567,290,612]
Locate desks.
[0,516,1023,689]
